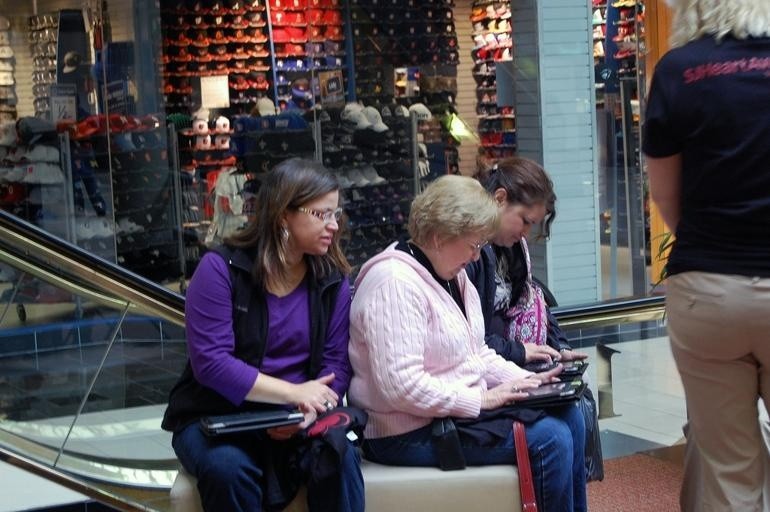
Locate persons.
[161,158,366,512]
[642,0,769,512]
[464,158,604,485]
[72,158,106,220]
[347,173,587,512]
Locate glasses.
[469,240,489,252]
[297,206,342,224]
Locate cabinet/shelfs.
[592,0,651,249]
[470,1,525,165]
[0,1,181,295]
[148,0,459,294]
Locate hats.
[1,113,173,285]
[230,152,268,230]
[232,101,432,157]
[163,1,458,104]
[470,1,518,164]
[62,51,82,74]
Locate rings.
[540,344,544,350]
[511,384,517,391]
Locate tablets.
[536,358,586,374]
[518,378,586,401]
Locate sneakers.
[321,143,412,290]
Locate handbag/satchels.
[505,238,548,345]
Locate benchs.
[170,455,522,512]
